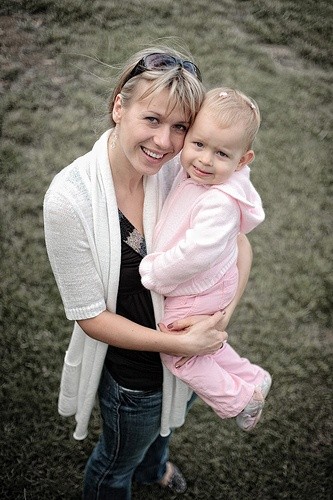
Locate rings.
[220,342,224,350]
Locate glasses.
[121,53,201,88]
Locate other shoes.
[261,371,273,398]
[237,389,265,432]
[164,465,187,495]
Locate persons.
[43,47,252,500]
[139,87,271,432]
